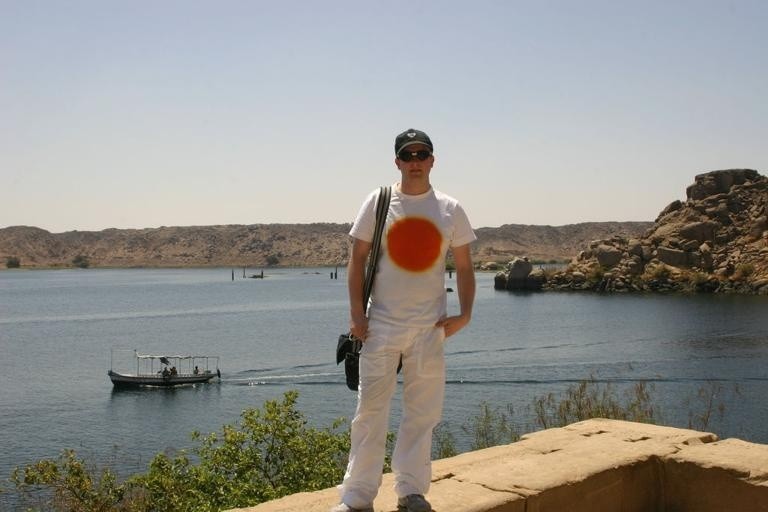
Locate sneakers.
[397,493,436,512]
[330,500,375,512]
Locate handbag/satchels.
[334,329,405,391]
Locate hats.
[394,127,434,158]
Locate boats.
[108,371,220,387]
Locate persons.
[193,366,200,374]
[333,127,480,512]
[170,366,177,375]
[161,366,170,376]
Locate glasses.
[398,149,432,163]
